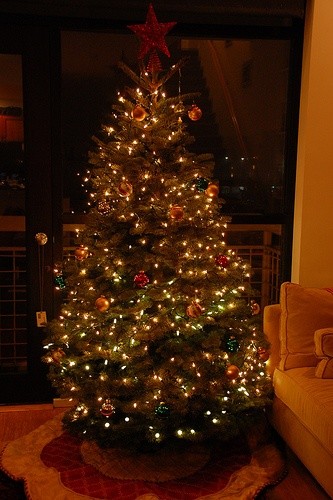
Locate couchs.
[262,303,333,500]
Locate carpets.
[1,410,289,499]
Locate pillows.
[279,281,333,373]
